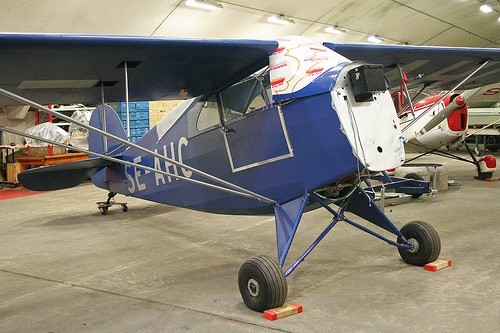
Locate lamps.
[367,36,385,45]
[267,12,295,27]
[323,25,348,36]
[184,0,224,11]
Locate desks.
[14,153,95,192]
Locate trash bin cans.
[7,163,20,183]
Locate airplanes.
[380,81,500,199]
[0,31,500,313]
